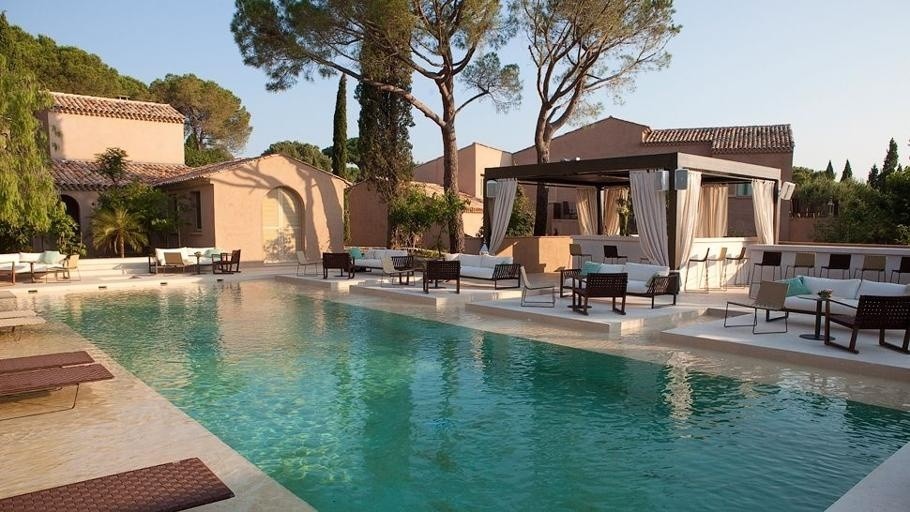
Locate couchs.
[0,252,68,283]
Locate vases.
[820,293,832,298]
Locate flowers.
[817,288,834,296]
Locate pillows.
[855,278,909,301]
[481,254,514,269]
[645,271,670,288]
[363,249,385,260]
[802,275,862,300]
[500,260,509,265]
[622,262,670,282]
[774,276,813,297]
[350,248,363,259]
[580,260,603,277]
[458,252,482,268]
[385,250,408,258]
[444,253,458,264]
[598,263,625,276]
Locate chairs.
[146,245,243,278]
[322,252,355,279]
[0,362,116,409]
[53,252,84,280]
[391,254,433,285]
[380,256,404,287]
[724,280,789,335]
[0,291,47,342]
[423,260,461,294]
[1,351,95,391]
[571,272,628,316]
[519,265,555,308]
[823,294,909,355]
[295,250,318,276]
[0,456,236,511]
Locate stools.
[890,254,909,284]
[700,247,728,292]
[819,252,852,280]
[683,247,710,293]
[602,244,628,265]
[748,250,783,298]
[567,243,592,270]
[726,247,750,289]
[853,254,887,283]
[785,251,816,281]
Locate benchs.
[560,262,682,309]
[765,275,909,323]
[437,252,520,289]
[352,248,414,275]
